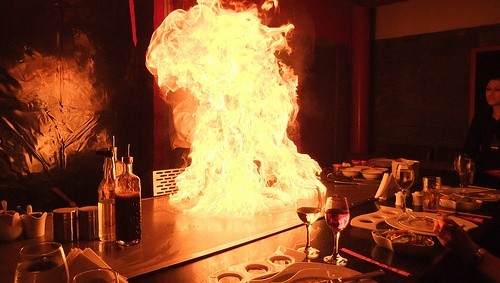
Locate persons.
[460,77,500,154]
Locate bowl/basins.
[341,167,362,178]
[372,229,434,254]
[439,194,483,212]
[361,170,382,181]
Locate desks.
[0,177,500,283]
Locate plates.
[385,213,479,236]
[441,188,499,202]
[250,262,369,283]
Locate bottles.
[98,156,115,242]
[115,156,142,247]
[110,146,126,177]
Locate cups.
[14,243,70,283]
[54,207,79,243]
[73,268,118,283]
[423,176,441,213]
[460,161,476,187]
[81,206,100,242]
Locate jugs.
[0,200,22,241]
[20,205,47,242]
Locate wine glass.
[394,169,414,211]
[454,152,471,187]
[323,194,350,266]
[297,184,322,259]
